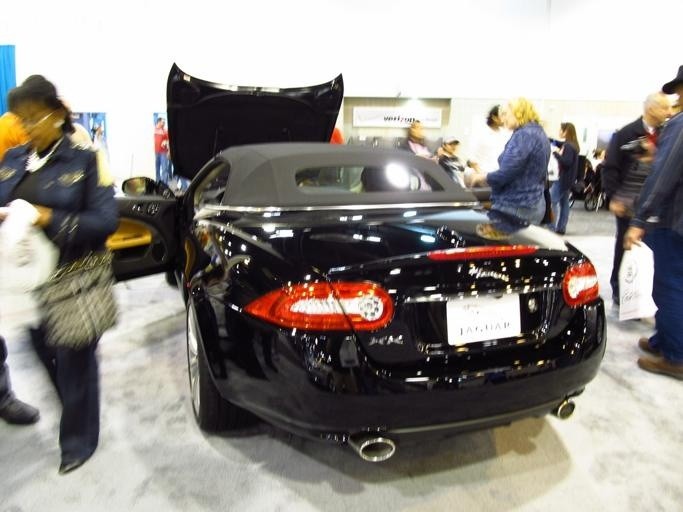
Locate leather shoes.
[0,398,41,425]
[56,439,99,475]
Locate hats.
[661,65,682,94]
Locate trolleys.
[567,155,605,211]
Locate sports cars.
[106,61,607,461]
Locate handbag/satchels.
[23,250,118,352]
[618,240,658,322]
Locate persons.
[0,74,117,476]
[154,117,170,187]
[396,98,581,237]
[583,65,683,384]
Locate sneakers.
[637,356,682,379]
[638,337,664,357]
